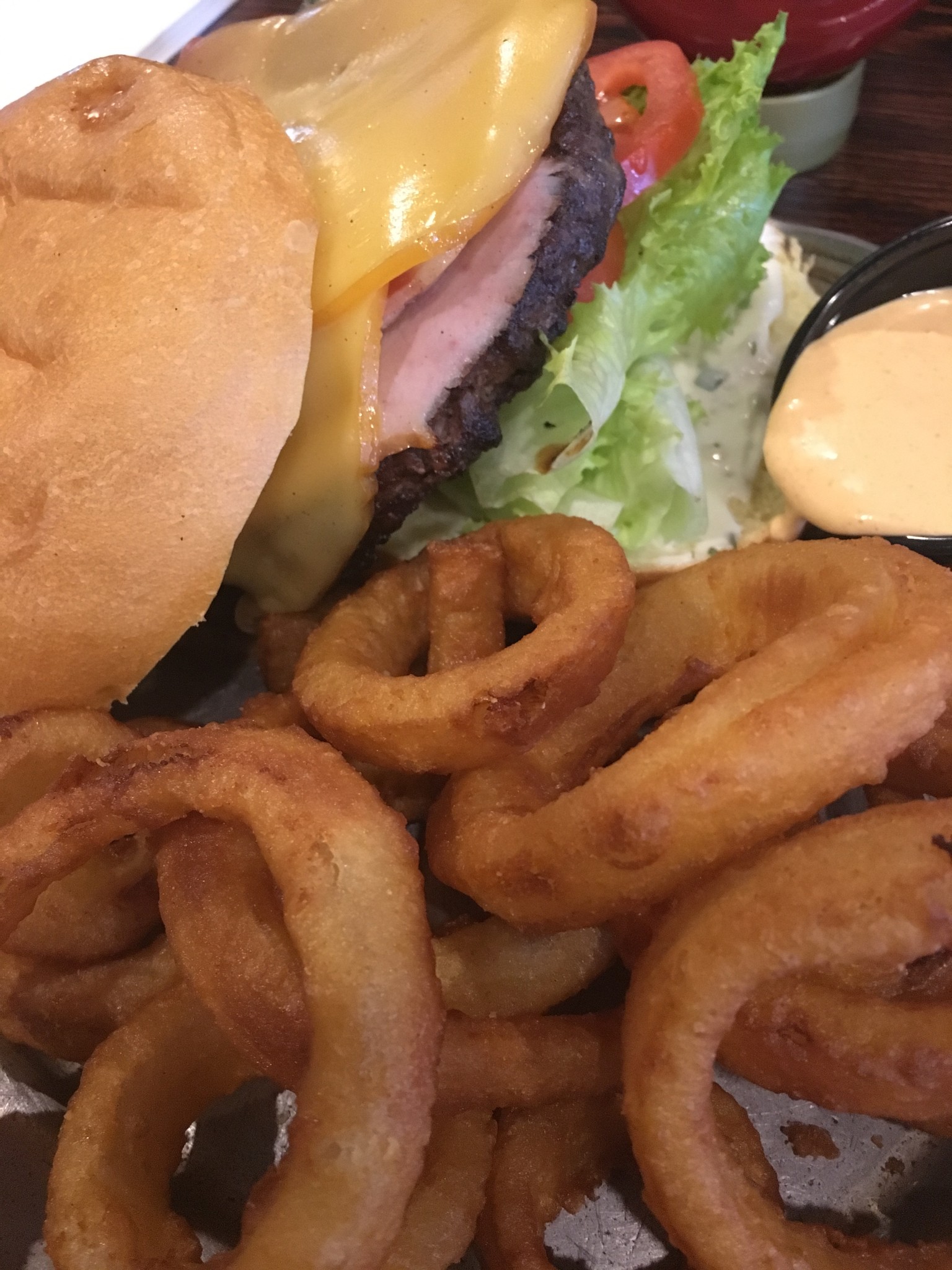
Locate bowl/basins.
[756,55,867,171]
[770,214,951,570]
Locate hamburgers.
[0,2,832,735]
